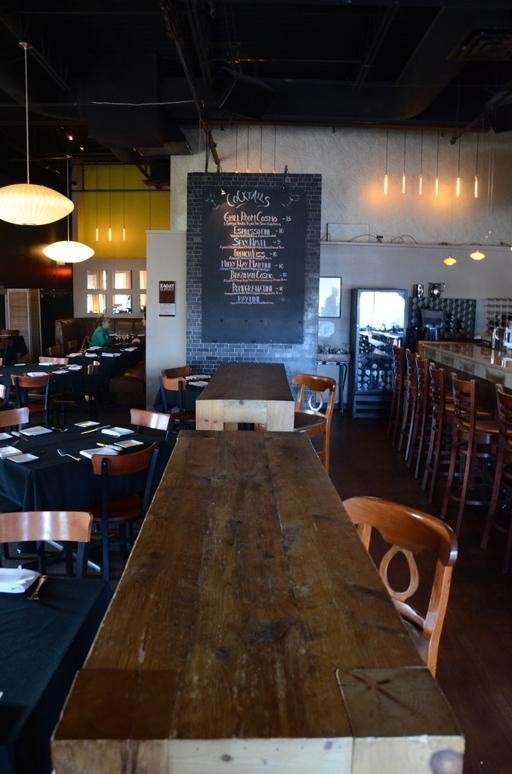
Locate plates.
[8,452,39,464]
[114,438,143,448]
[74,419,100,428]
[0,432,13,440]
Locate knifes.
[96,442,123,452]
[12,430,29,441]
[80,423,112,436]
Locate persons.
[91,315,112,347]
[132,312,148,394]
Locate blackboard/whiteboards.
[201,186,307,344]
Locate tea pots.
[427,281,445,297]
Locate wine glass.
[113,329,131,345]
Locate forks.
[56,448,81,461]
[53,425,69,433]
[3,440,21,447]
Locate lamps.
[381,124,483,200]
[40,153,97,265]
[0,35,75,228]
[92,154,126,242]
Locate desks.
[47,426,471,774]
[193,359,297,435]
[1,571,114,774]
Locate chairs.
[1,311,175,594]
[253,372,338,479]
[339,494,462,680]
[158,364,212,440]
[387,339,512,555]
[359,320,404,390]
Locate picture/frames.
[317,275,343,319]
